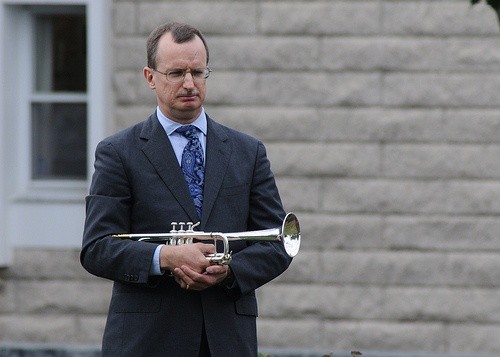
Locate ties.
[175,125,205,219]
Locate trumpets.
[111,212,301,276]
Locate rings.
[186,285,189,290]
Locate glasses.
[148,66,212,82]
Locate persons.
[79,22,294,357]
